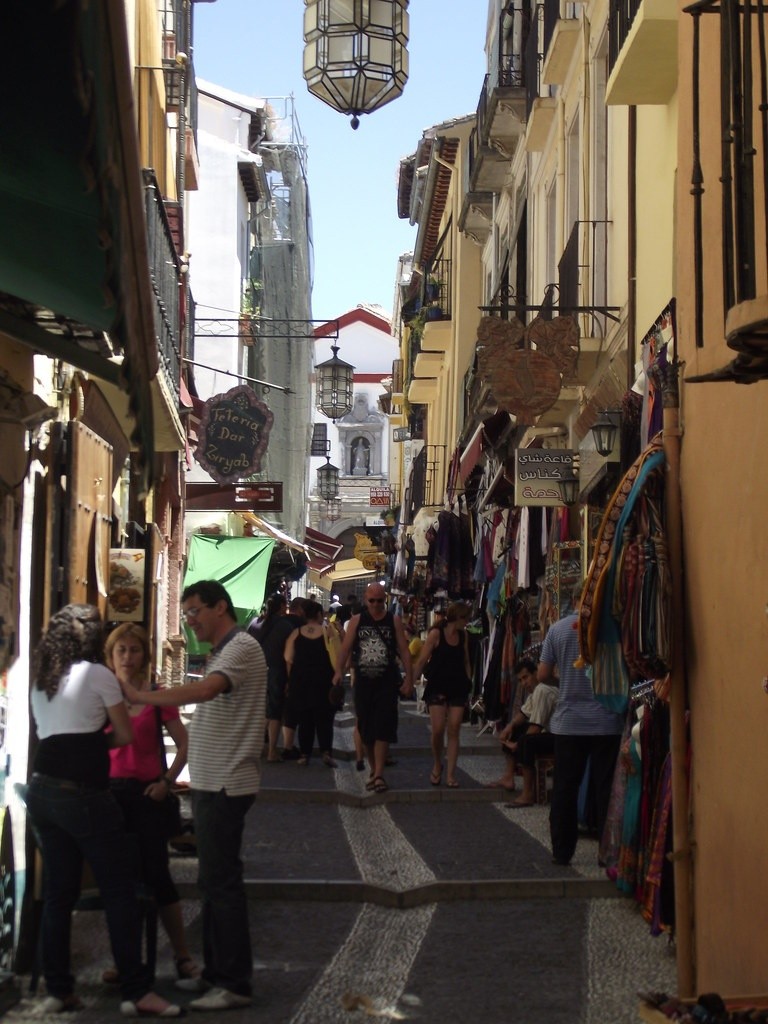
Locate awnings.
[304,526,344,579]
[237,512,309,553]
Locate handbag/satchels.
[395,673,406,693]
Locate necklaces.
[127,680,142,709]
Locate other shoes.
[171,953,204,983]
[326,758,338,769]
[281,747,302,760]
[268,749,284,764]
[173,975,210,993]
[298,757,309,766]
[322,622,352,673]
[384,759,398,767]
[356,760,366,772]
[187,986,251,1012]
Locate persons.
[247,594,424,772]
[332,583,413,792]
[405,602,472,789]
[102,622,201,985]
[0,614,19,683]
[353,438,368,468]
[536,579,628,864]
[25,603,189,1018]
[491,658,559,808]
[426,494,472,602]
[119,580,268,1011]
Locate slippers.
[503,799,533,809]
[484,779,515,792]
[445,777,459,789]
[431,765,444,785]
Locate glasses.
[367,597,385,603]
[182,603,208,622]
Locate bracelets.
[161,776,171,785]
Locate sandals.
[373,776,389,793]
[44,993,96,1014]
[118,997,189,1019]
[366,773,375,791]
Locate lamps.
[590,407,621,459]
[310,438,342,524]
[303,0,410,132]
[191,315,354,427]
